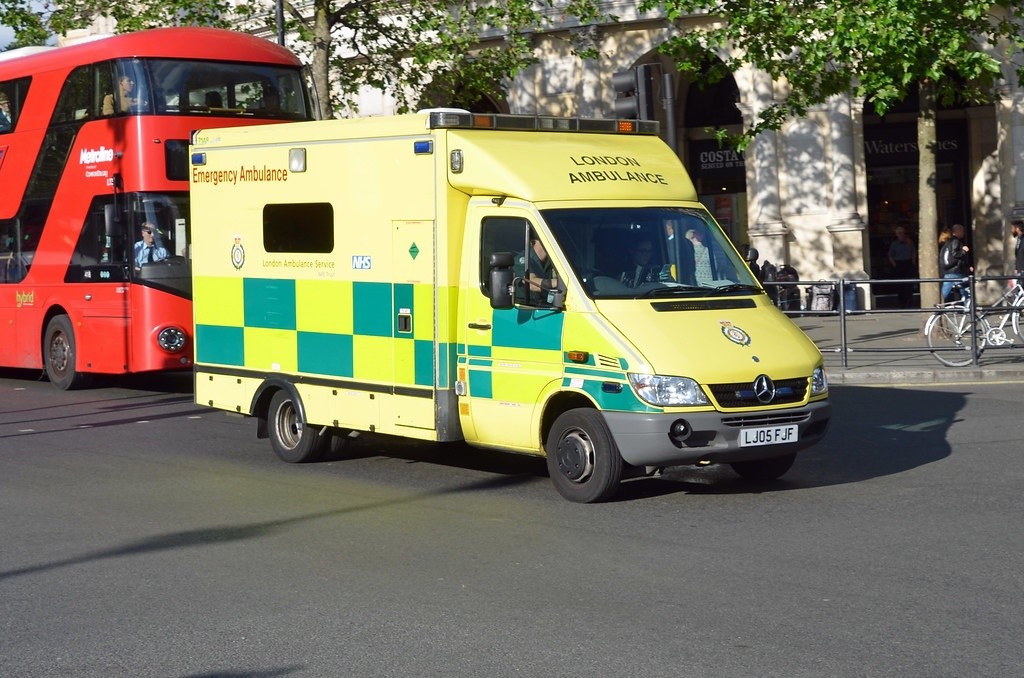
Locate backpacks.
[939,240,958,271]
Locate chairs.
[494,225,524,256]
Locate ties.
[147,246,154,263]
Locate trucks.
[189,111,832,505]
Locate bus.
[1,26,321,390]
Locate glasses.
[146,229,153,233]
[896,231,906,234]
[0,101,11,106]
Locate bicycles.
[925,274,1024,367]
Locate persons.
[938,220,1024,321]
[666,220,718,287]
[102,72,148,116]
[205,91,222,108]
[616,233,662,288]
[888,227,916,309]
[0,91,16,132]
[134,221,171,268]
[263,85,282,109]
[513,233,558,308]
